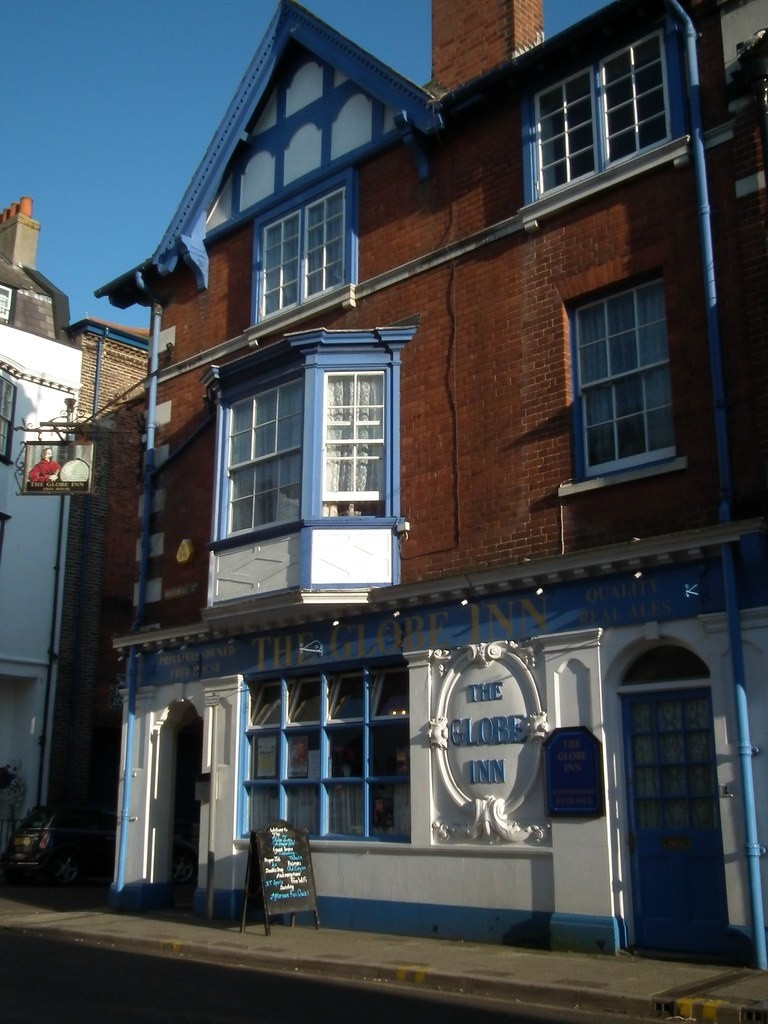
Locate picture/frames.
[289,735,308,779]
[254,733,279,779]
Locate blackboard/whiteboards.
[254,819,318,916]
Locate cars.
[0,807,199,887]
